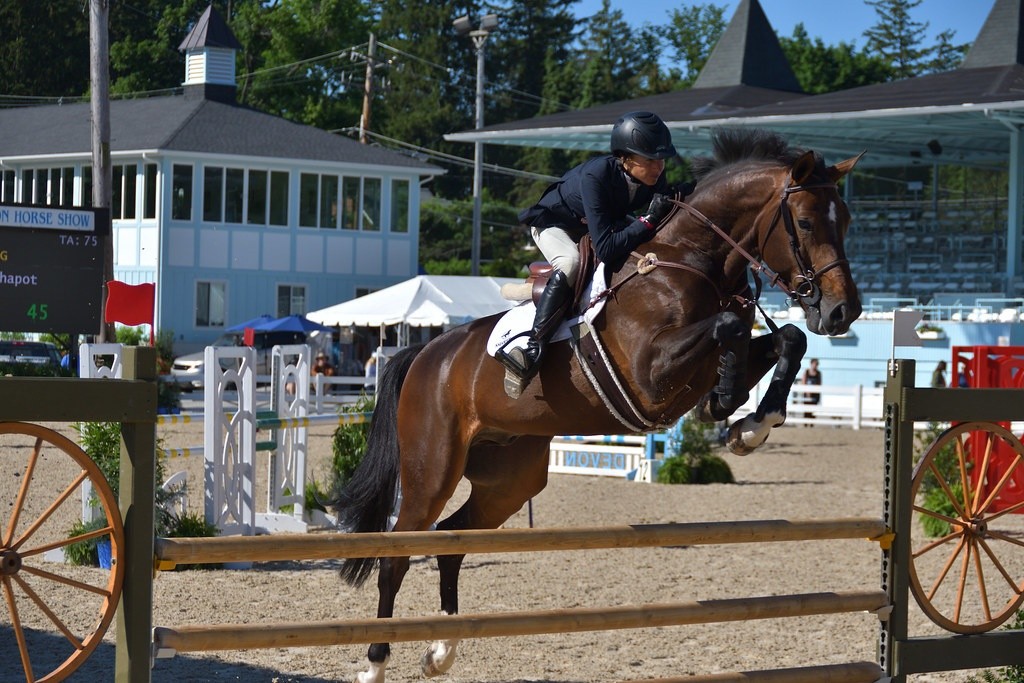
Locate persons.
[286,352,334,394]
[504,111,697,400]
[932,361,968,388]
[365,352,377,395]
[61,354,69,370]
[801,358,822,427]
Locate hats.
[316,352,329,361]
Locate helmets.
[611,112,677,160]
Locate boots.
[504,270,573,401]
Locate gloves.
[645,191,674,221]
[669,179,699,197]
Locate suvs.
[170,330,319,393]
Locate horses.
[310,121,864,682]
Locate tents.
[306,275,526,346]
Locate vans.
[0,338,63,368]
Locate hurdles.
[1,329,1024,682]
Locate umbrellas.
[224,313,338,390]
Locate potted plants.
[63,505,223,568]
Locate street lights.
[450,13,499,276]
